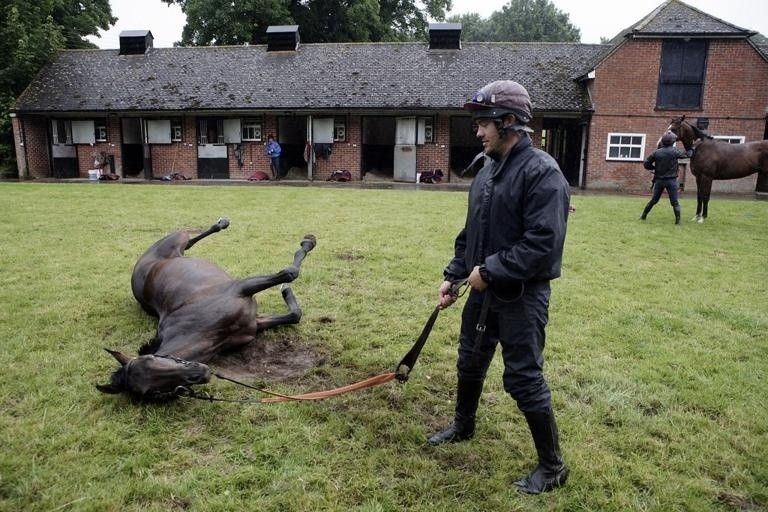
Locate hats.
[464,79,533,126]
[661,129,679,145]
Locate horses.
[97,217,316,404]
[656,114,767,224]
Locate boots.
[641,200,652,221]
[428,377,484,447]
[674,206,682,226]
[510,408,571,495]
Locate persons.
[265,135,281,181]
[640,130,702,224]
[428,81,570,494]
